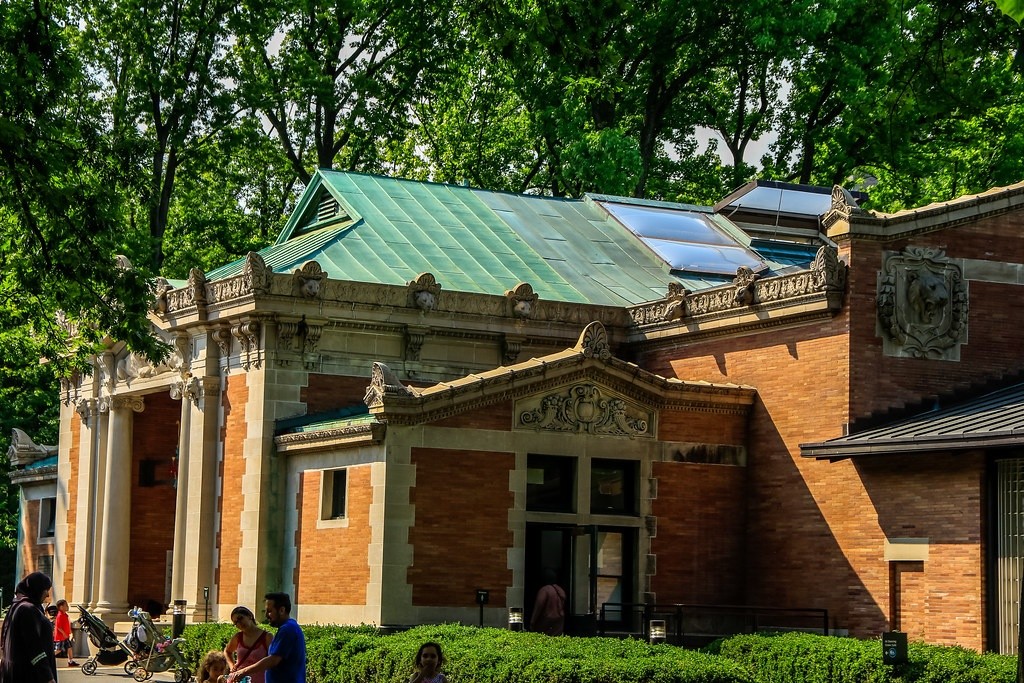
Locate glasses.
[233,613,249,628]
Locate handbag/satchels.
[564,604,575,623]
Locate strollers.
[75,604,140,676]
[127,606,192,683]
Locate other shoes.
[68,661,80,666]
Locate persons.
[409,642,447,683]
[0,572,57,683]
[200,593,306,683]
[54,599,80,667]
[529,573,566,636]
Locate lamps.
[475,589,489,627]
[649,619,668,644]
[172,599,188,639]
[204,586,209,623]
[507,606,526,632]
